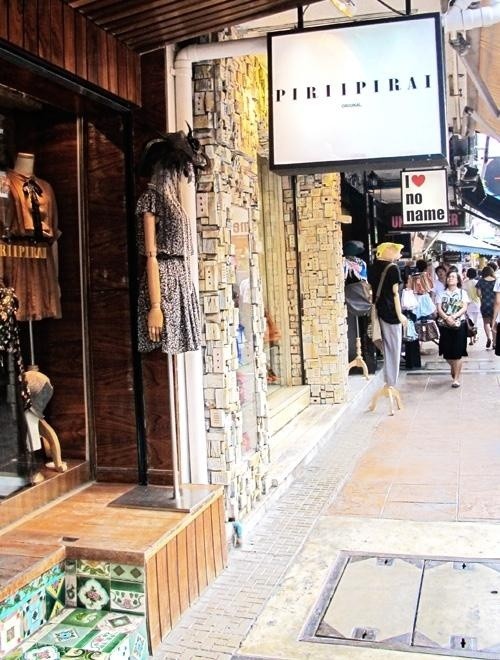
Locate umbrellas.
[451,367,460,387]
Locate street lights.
[465,318,477,337]
[471,287,479,303]
[371,304,384,354]
[346,283,371,311]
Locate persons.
[133,129,207,355]
[0,152,62,322]
[342,240,372,314]
[368,242,500,388]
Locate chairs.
[469,337,478,345]
[486,339,496,349]
